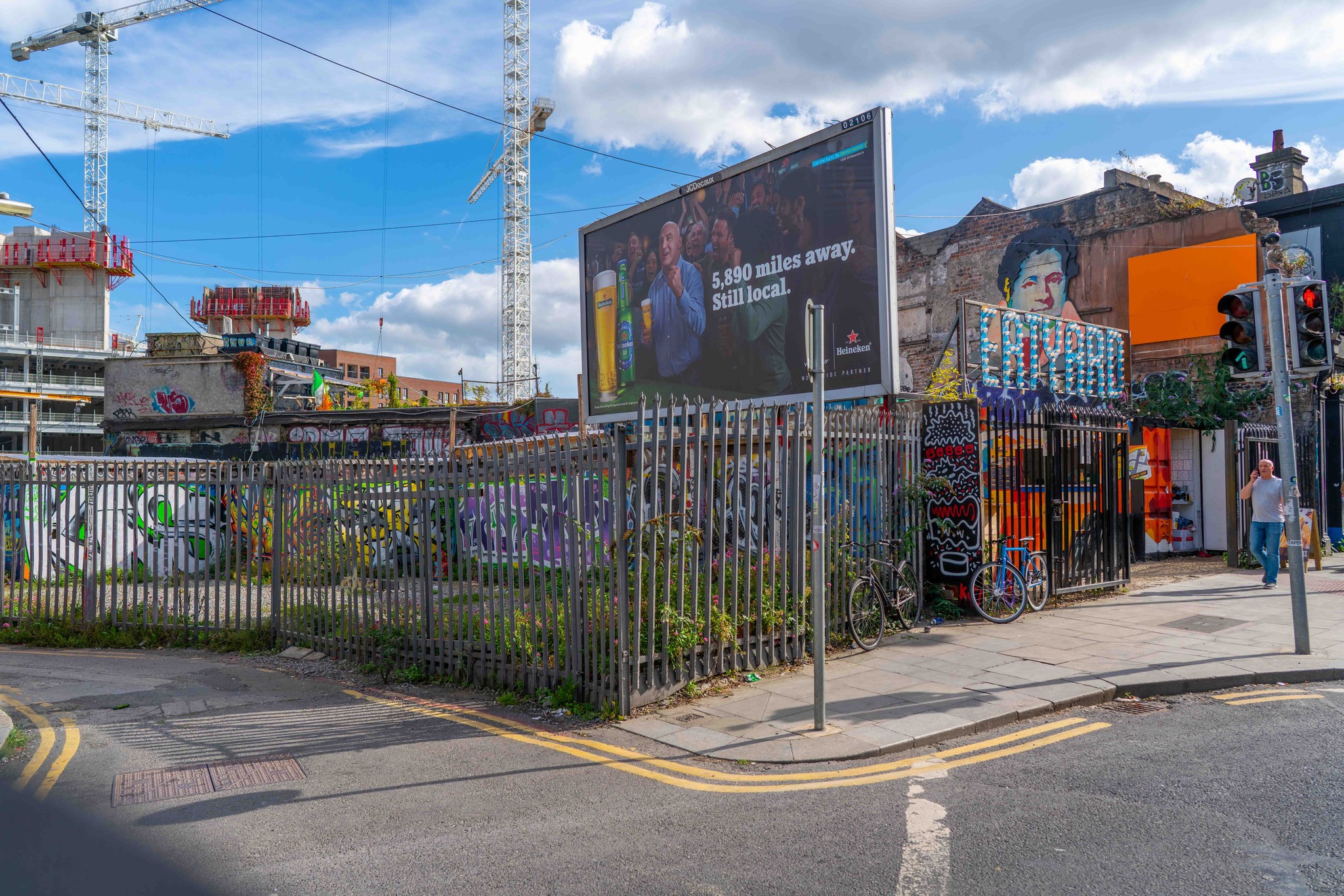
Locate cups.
[593,269,619,403]
[641,298,653,341]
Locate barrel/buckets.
[1172,511,1180,529]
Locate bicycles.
[837,538,923,652]
[968,535,1050,624]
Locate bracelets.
[1250,481,1254,483]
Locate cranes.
[0,71,232,142]
[8,0,232,230]
[464,0,557,406]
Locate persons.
[1240,459,1285,589]
[585,139,879,396]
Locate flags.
[311,368,334,412]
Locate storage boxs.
[1173,529,1194,550]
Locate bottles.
[615,259,636,388]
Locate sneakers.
[1263,582,1278,589]
[1262,572,1268,583]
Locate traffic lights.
[1216,287,1267,379]
[1286,279,1334,374]
